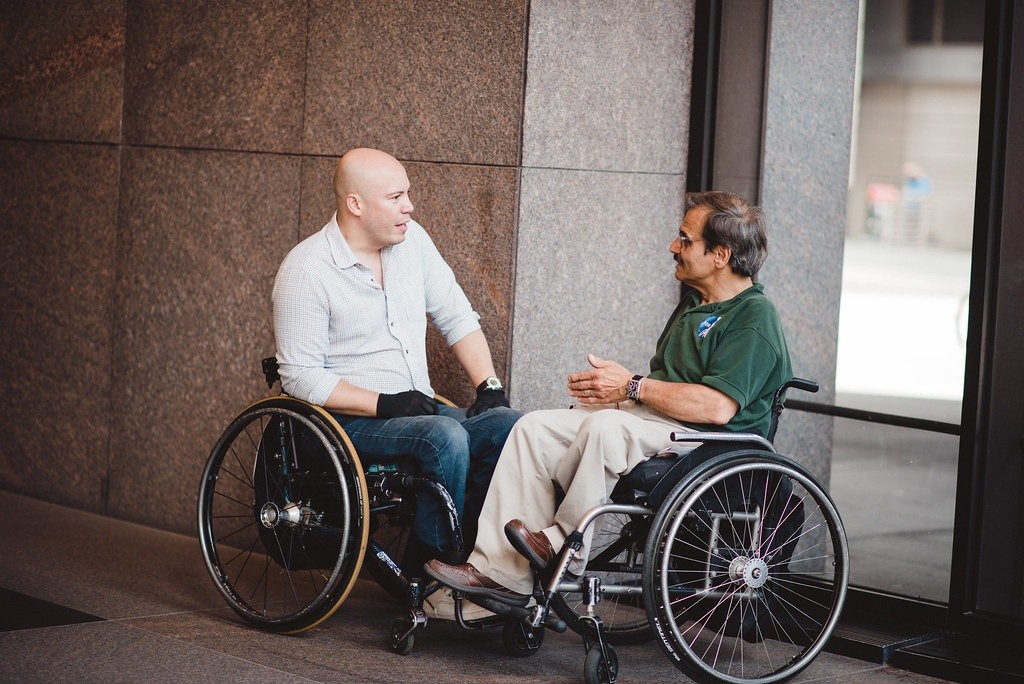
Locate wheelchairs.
[452,372,849,684]
[195,356,543,653]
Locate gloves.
[376,390,439,421]
[466,375,512,419]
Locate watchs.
[475,375,505,394]
[627,374,645,403]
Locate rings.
[586,389,591,396]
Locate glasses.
[679,232,705,248]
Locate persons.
[421,191,795,608]
[267,146,529,624]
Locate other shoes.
[423,586,498,621]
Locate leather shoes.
[423,559,537,607]
[505,518,581,582]
